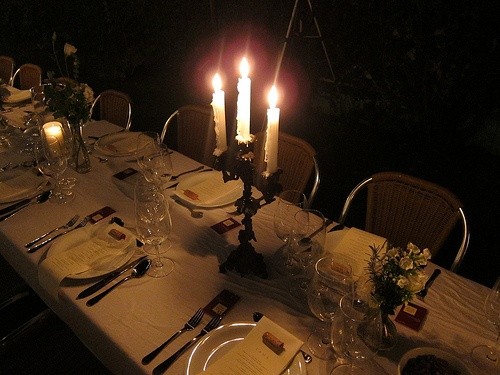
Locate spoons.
[170,195,203,218]
[0,189,53,223]
[168,166,205,182]
[86,259,152,306]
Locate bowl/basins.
[398,347,473,375]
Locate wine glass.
[0,108,79,206]
[326,291,382,375]
[286,208,326,300]
[274,189,310,274]
[470,280,500,373]
[308,255,353,360]
[133,131,174,277]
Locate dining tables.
[0,82,500,375]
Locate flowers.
[44,41,95,166]
[365,240,432,328]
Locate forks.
[24,215,91,253]
[152,310,224,375]
[142,307,207,365]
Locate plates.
[185,321,307,375]
[3,89,33,102]
[47,223,136,279]
[175,170,244,207]
[94,131,153,157]
[0,163,51,203]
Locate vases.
[66,120,93,174]
[371,297,398,351]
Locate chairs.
[0,56,470,271]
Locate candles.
[234,58,252,142]
[211,69,227,154]
[265,88,281,176]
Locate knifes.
[76,255,149,298]
[163,169,213,191]
